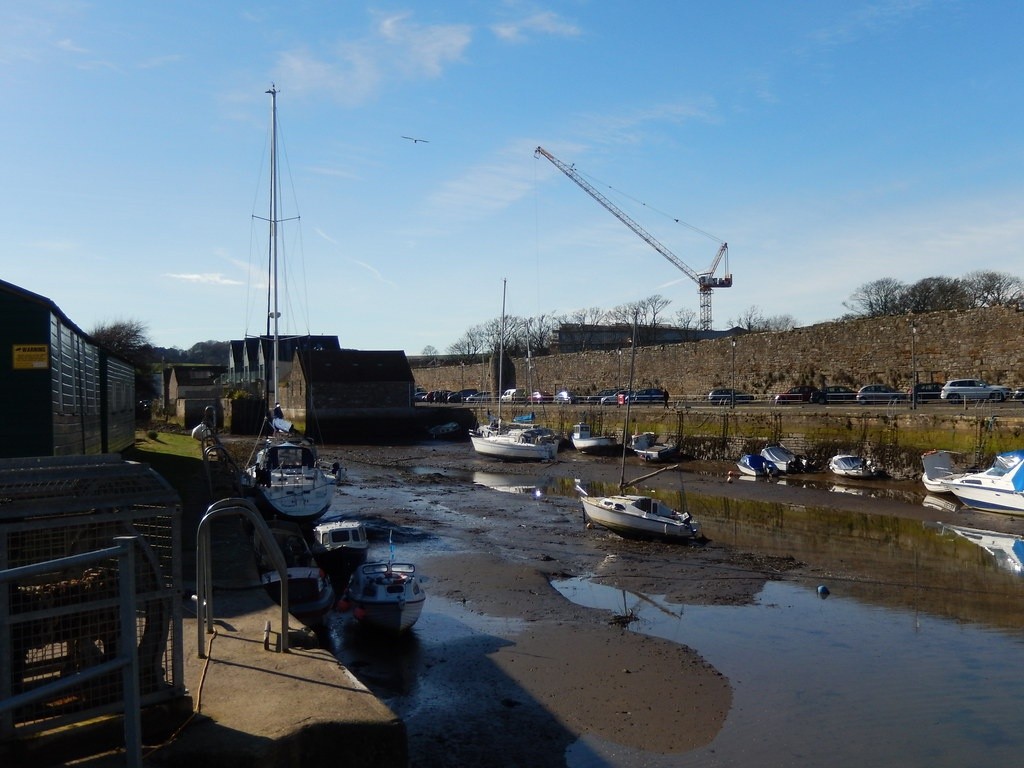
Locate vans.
[501,388,524,404]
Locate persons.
[664,389,670,409]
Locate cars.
[809,385,858,406]
[415,388,478,404]
[856,384,909,404]
[774,385,819,404]
[585,387,635,406]
[1013,387,1024,401]
[527,391,554,405]
[908,382,946,404]
[466,391,493,402]
[625,388,665,403]
[553,391,577,404]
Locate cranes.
[533,147,734,331]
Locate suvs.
[940,379,1013,405]
[709,388,754,405]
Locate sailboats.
[920,519,1024,577]
[254,80,344,529]
[467,280,559,464]
[573,308,703,540]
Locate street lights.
[616,347,622,408]
[523,354,529,403]
[731,337,741,409]
[910,320,920,410]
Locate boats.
[253,519,426,649]
[920,491,962,517]
[739,454,780,479]
[759,444,791,474]
[828,485,870,497]
[919,450,974,492]
[828,453,878,479]
[572,422,618,455]
[632,423,676,463]
[736,475,766,481]
[938,450,1023,516]
[469,471,548,497]
